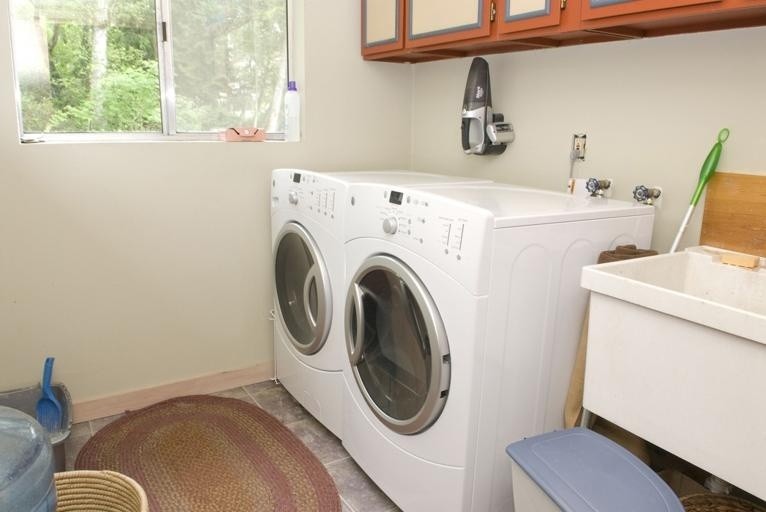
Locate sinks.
[579,246,765,502]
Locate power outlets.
[572,131,585,161]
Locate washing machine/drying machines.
[273,168,493,439]
[341,183,658,512]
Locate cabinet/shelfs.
[581,0,766,40]
[360,0,545,64]
[495,0,636,46]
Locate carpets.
[75,391,343,512]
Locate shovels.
[35,357,64,433]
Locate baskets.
[51,471,147,511]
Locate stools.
[505,421,688,512]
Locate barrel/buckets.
[0,401,57,511]
